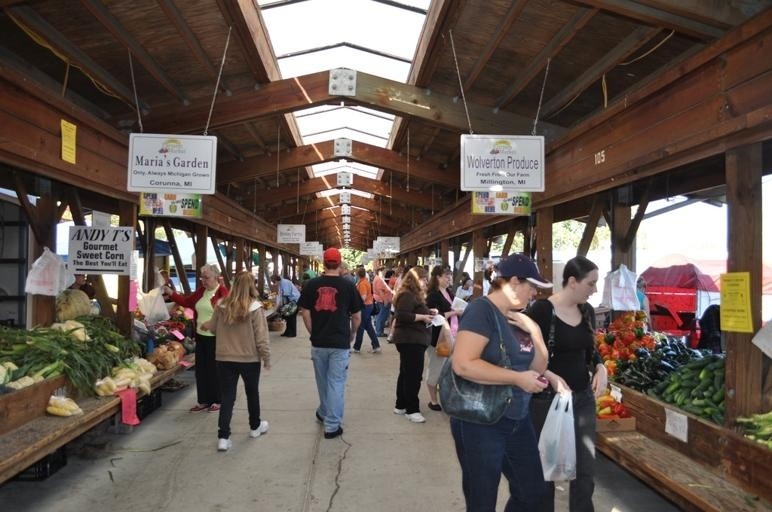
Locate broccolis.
[0,362,33,389]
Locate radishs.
[94,368,153,396]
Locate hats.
[324,248,341,264]
[497,252,554,288]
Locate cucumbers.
[648,355,726,427]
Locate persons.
[636,276,650,332]
[339,262,474,423]
[296,247,364,439]
[525,255,607,511]
[66,247,324,451]
[450,252,554,511]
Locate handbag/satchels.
[280,301,298,317]
[532,299,556,398]
[371,298,379,315]
[436,297,513,424]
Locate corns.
[47,397,77,417]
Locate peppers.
[594,395,631,419]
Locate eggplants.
[616,338,704,394]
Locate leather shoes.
[428,402,441,410]
[190,406,219,413]
[316,411,343,438]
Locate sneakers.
[217,438,232,450]
[351,335,394,353]
[393,406,425,423]
[250,421,268,438]
